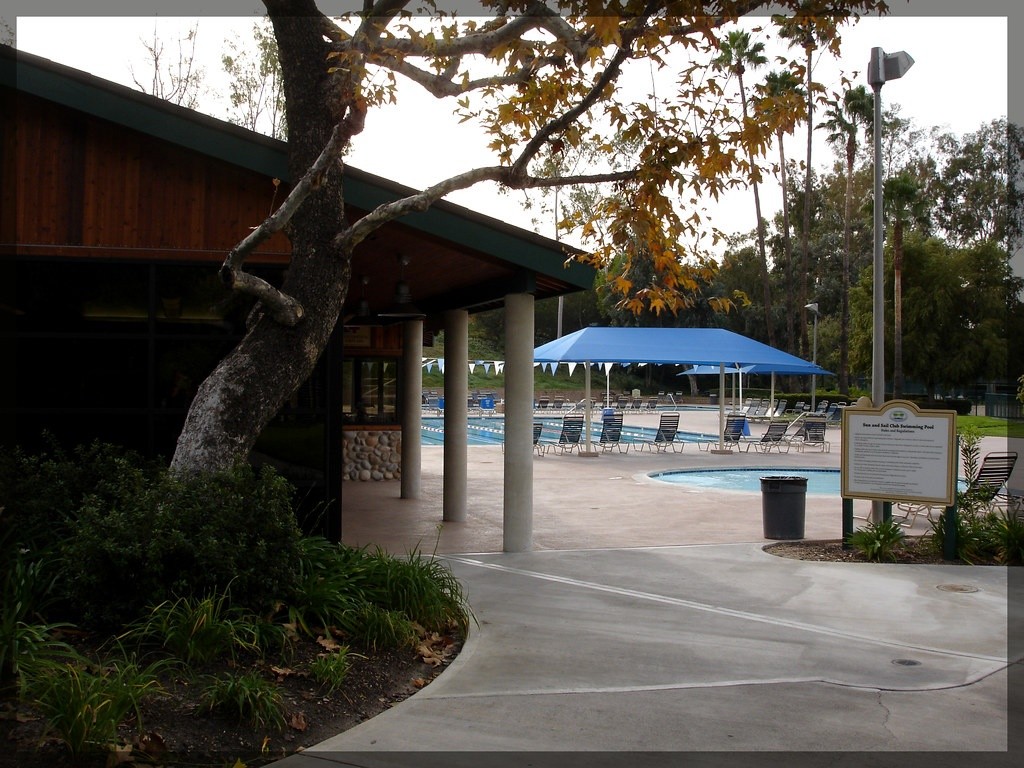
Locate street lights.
[866,48,916,529]
[708,297,726,453]
[806,303,819,412]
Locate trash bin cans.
[709,394,717,405]
[759,475,809,540]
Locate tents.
[533,327,836,452]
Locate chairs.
[422,390,857,457]
[853,451,1024,528]
